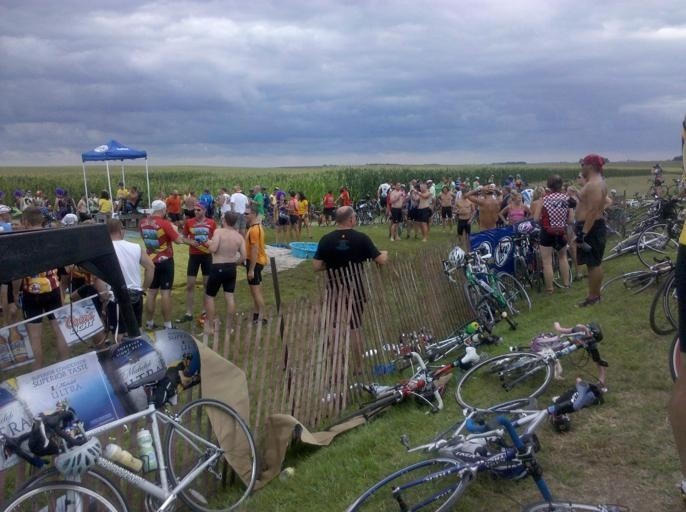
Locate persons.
[568,154,610,309]
[650,162,664,197]
[309,204,389,385]
[53,399,83,436]
[665,118,686,505]
[164,351,200,394]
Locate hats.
[152,200,167,211]
[583,154,604,171]
[192,201,208,210]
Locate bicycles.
[302,200,339,227]
[0,366,258,512]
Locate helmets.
[547,173,564,189]
[489,465,529,480]
[518,222,533,233]
[449,246,465,264]
[54,436,102,475]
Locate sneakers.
[164,325,177,329]
[145,322,158,329]
[574,297,599,308]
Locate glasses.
[244,212,254,215]
[191,208,200,211]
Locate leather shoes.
[174,315,192,323]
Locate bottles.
[136,428,159,474]
[106,443,145,473]
[460,444,487,457]
[466,321,479,334]
